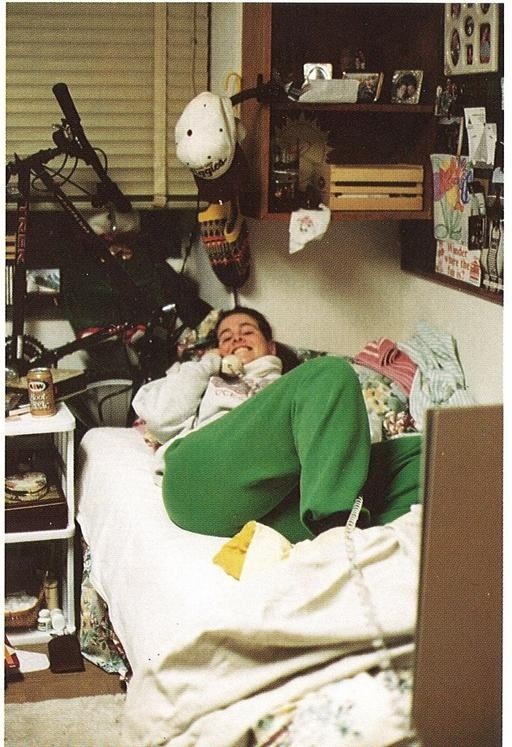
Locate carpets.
[4,693,127,746]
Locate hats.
[175,91,248,192]
[197,194,251,289]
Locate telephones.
[220,355,242,376]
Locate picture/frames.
[339,72,384,103]
[443,3,499,76]
[390,69,424,104]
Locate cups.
[271,134,302,210]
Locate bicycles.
[7,80,204,432]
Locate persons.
[131,302,426,544]
[396,83,407,97]
[406,82,416,97]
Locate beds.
[77,421,426,747]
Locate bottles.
[37,609,66,632]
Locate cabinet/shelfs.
[6,399,76,648]
[240,2,442,221]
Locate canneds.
[27,367,57,418]
